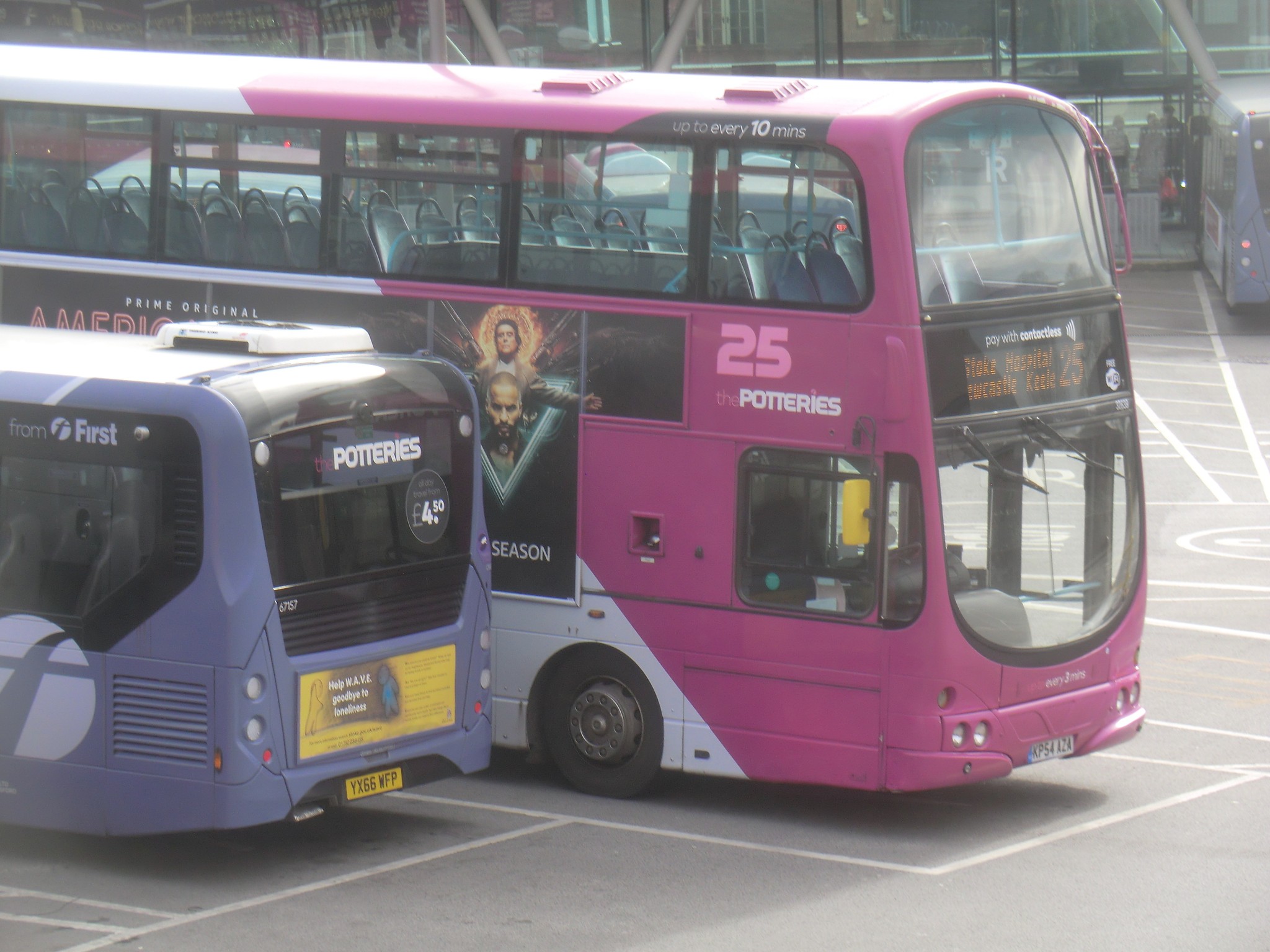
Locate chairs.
[1,160,985,310]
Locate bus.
[0,317,493,836]
[0,41,1151,796]
[1196,85,1270,317]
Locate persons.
[466,319,602,483]
[1110,104,1184,217]
